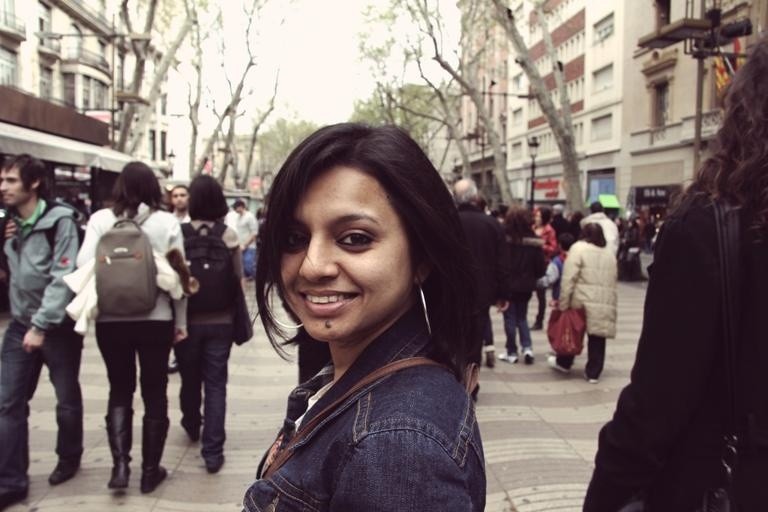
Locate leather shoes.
[50,464,78,483]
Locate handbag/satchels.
[547,307,587,356]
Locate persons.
[0,153,83,511]
[243,123,486,512]
[77,161,190,492]
[232,176,667,385]
[175,176,241,472]
[582,32,768,508]
[166,184,191,371]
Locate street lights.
[33,13,154,152]
[166,150,176,180]
[528,136,540,211]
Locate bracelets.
[31,324,46,337]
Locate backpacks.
[95,207,161,316]
[178,221,234,314]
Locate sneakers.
[523,350,534,364]
[583,373,598,384]
[498,353,518,363]
[547,356,570,373]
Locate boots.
[484,344,495,368]
[105,407,133,488]
[141,414,168,492]
